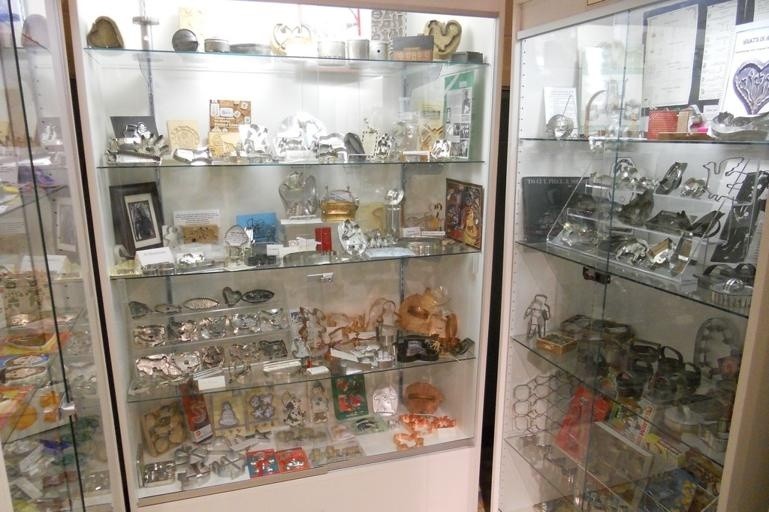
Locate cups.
[319,39,388,61]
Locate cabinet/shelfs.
[481,1,768,512]
[67,0,507,512]
[0,0,128,512]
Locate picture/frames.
[51,189,81,262]
[109,181,166,257]
[444,177,483,250]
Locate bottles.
[1,14,24,48]
